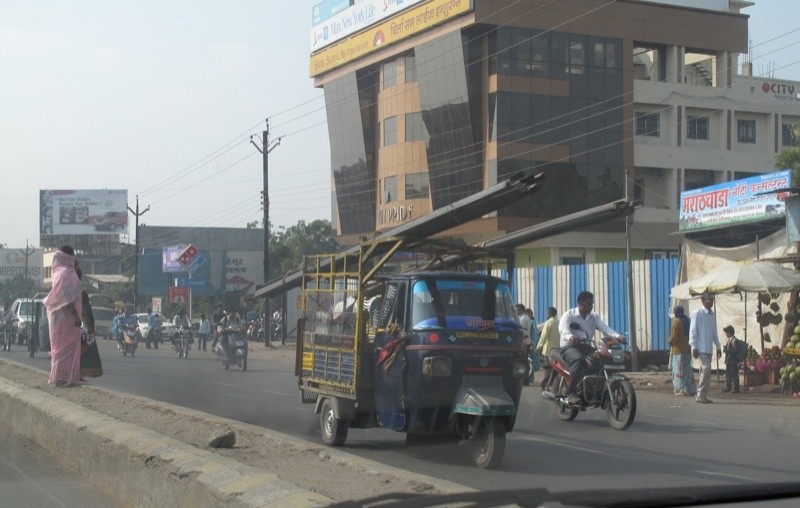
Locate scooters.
[212,322,249,373]
[169,325,194,360]
[118,319,140,358]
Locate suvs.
[9,298,37,345]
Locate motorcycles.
[541,322,637,431]
[294,234,533,470]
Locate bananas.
[756,294,782,342]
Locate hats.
[723,326,734,332]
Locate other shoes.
[720,389,731,392]
[674,391,685,397]
[568,392,581,405]
[731,387,740,393]
[695,396,712,403]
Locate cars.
[91,306,116,340]
[131,313,176,343]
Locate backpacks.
[730,338,748,362]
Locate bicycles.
[246,318,282,343]
[0,316,20,352]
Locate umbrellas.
[670,260,800,390]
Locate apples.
[746,345,782,363]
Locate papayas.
[780,364,800,379]
[785,319,800,349]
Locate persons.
[43,245,103,387]
[667,305,695,396]
[722,325,739,393]
[172,308,245,352]
[516,303,559,390]
[0,303,19,328]
[689,293,722,403]
[114,309,139,347]
[558,290,626,403]
[146,308,164,349]
